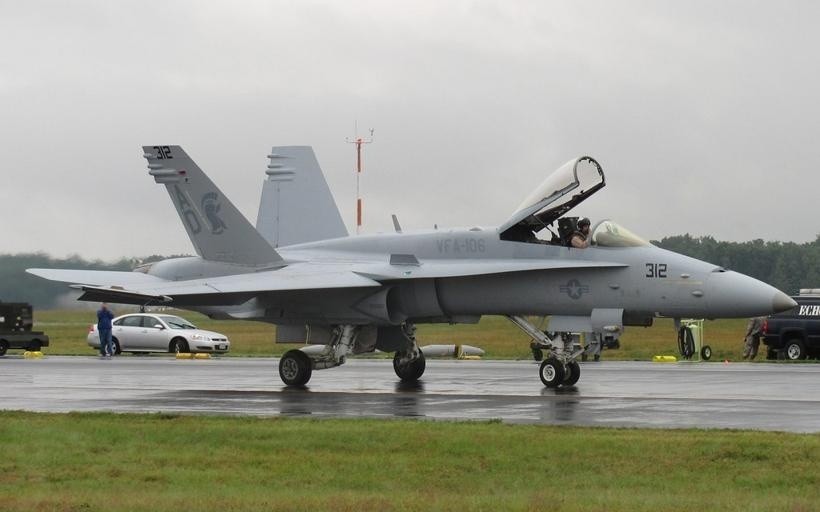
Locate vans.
[762,288,820,361]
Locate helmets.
[577,217,590,228]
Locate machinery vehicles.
[0,300,49,356]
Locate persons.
[565,217,592,248]
[741,317,764,359]
[98,302,115,356]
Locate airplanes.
[25,145,799,388]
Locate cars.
[87,312,230,355]
